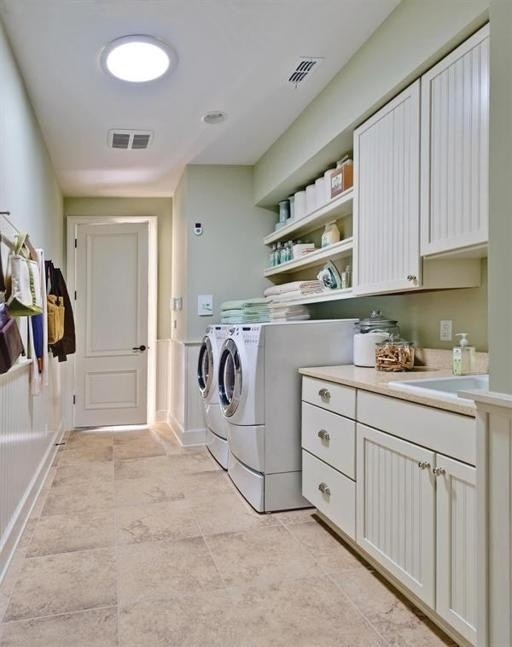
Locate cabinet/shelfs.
[357,96,419,291]
[258,188,356,307]
[354,424,477,636]
[297,376,355,544]
[430,38,489,246]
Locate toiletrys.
[452,332,476,376]
[342,266,350,289]
[271,240,301,267]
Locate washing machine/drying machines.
[196,325,228,477]
[218,315,361,515]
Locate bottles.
[341,265,352,288]
[352,310,401,367]
[375,332,416,372]
[270,239,302,266]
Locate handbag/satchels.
[47,295,65,344]
[0,306,23,374]
[4,255,43,316]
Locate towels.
[262,281,319,298]
[273,306,309,322]
[220,300,269,325]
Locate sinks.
[388,373,489,408]
